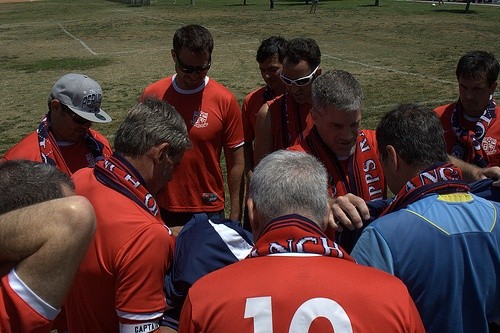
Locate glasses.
[65,109,91,124]
[280,65,321,86]
[174,50,211,72]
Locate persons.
[348,104,500,333]
[438,0,446,7]
[255,37,324,172]
[0,73,114,177]
[431,50,500,185]
[243,35,290,234]
[72,94,192,333]
[309,0,318,13]
[139,26,247,239]
[177,147,425,333]
[1,158,97,333]
[285,69,500,231]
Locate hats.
[51,74,112,122]
